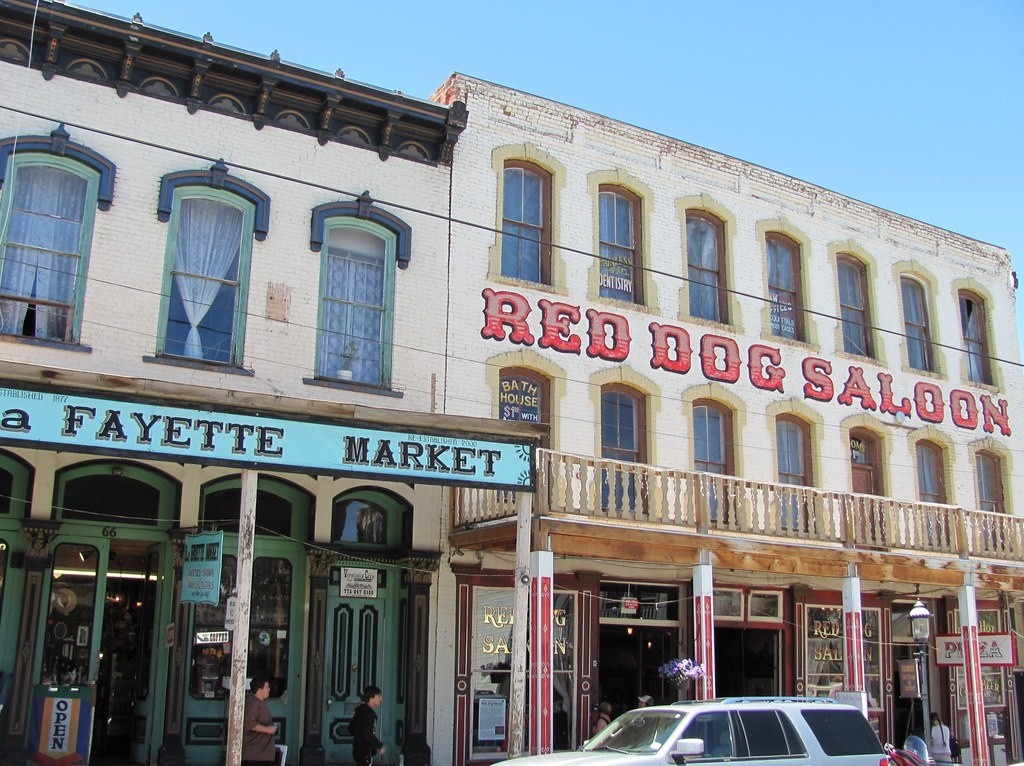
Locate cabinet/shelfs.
[41,604,154,756]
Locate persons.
[347,686,385,766]
[241,675,278,766]
[637,695,654,708]
[929,712,951,762]
[596,702,612,734]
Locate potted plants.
[335,340,358,380]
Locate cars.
[474,690,508,702]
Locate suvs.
[490,696,889,766]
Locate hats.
[639,695,654,705]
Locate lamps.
[908,596,934,652]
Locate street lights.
[78,551,122,602]
[906,598,936,766]
